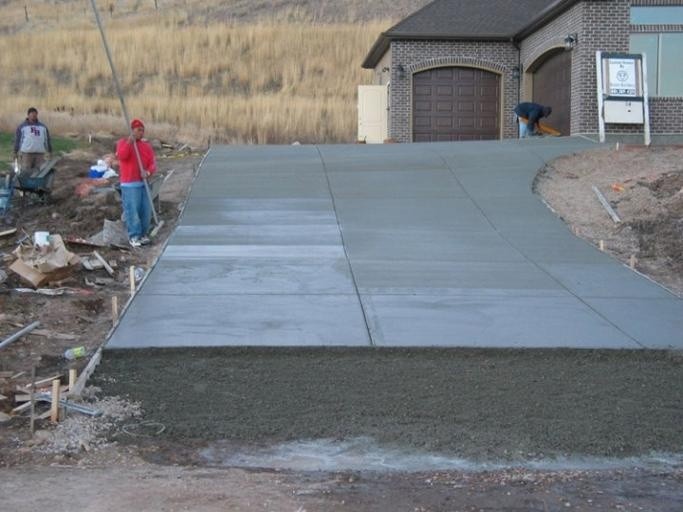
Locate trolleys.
[5,155,59,205]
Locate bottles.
[129,267,145,284]
[57,344,86,360]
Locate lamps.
[565,32,577,52]
[512,63,523,79]
[396,62,404,77]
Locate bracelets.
[147,171,151,177]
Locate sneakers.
[141,235,150,244]
[128,238,141,248]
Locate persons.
[14,108,52,173]
[116,120,156,247]
[515,102,552,138]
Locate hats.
[130,119,144,130]
